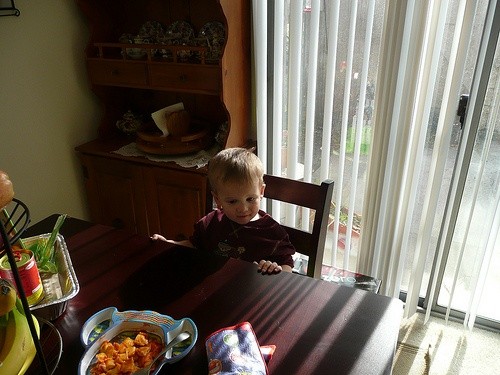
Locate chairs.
[262,174,334,278]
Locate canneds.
[0,250,45,307]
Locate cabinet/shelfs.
[73,0,251,240]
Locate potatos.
[95,332,163,375]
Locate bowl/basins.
[22,232,80,320]
[78,307,198,375]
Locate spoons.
[132,332,189,375]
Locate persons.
[149,147,295,273]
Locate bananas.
[0,279,40,375]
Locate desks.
[15,213,403,375]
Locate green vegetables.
[26,214,65,273]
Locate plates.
[24,318,62,375]
